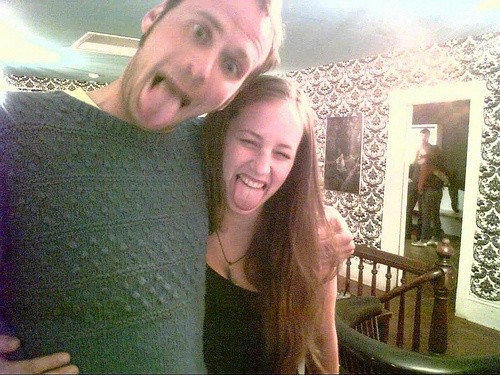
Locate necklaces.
[216,226,258,287]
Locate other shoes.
[411,241,427,249]
[425,241,438,248]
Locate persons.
[405,147,429,241]
[328,119,360,191]
[0,1,355,375]
[0,73,341,375]
[415,128,436,161]
[410,144,450,248]
